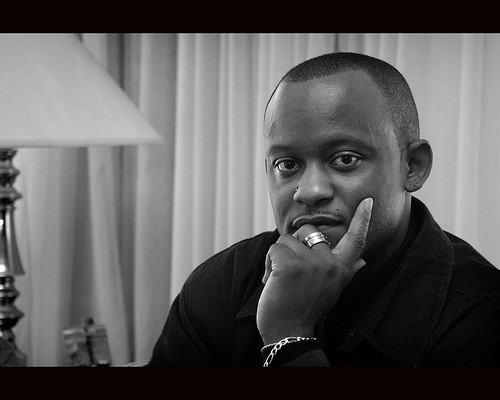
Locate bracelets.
[261,337,316,367]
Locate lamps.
[0,32,163,369]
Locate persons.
[147,52,499,400]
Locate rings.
[303,231,331,248]
